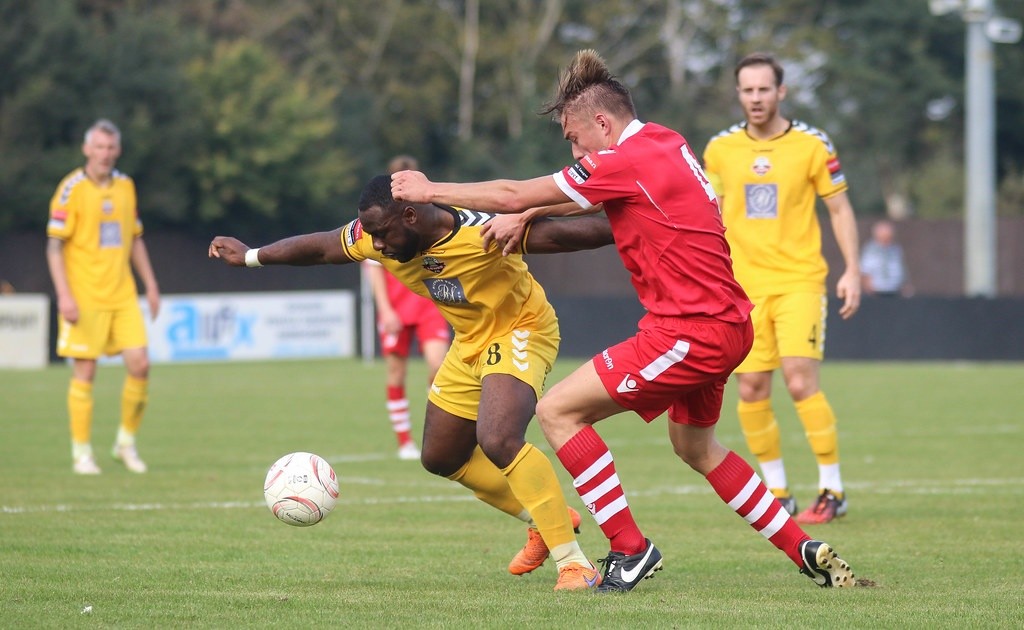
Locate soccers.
[265,452,340,526]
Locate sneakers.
[797,488,847,524]
[595,538,663,594]
[777,494,797,516]
[509,505,581,577]
[799,539,856,588]
[554,559,603,592]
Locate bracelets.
[244,248,263,267]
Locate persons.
[856,223,907,293]
[386,48,857,588]
[45,119,160,475]
[366,157,453,457]
[699,55,865,524]
[207,173,619,588]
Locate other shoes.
[112,445,147,475]
[399,443,422,460]
[73,451,101,476]
